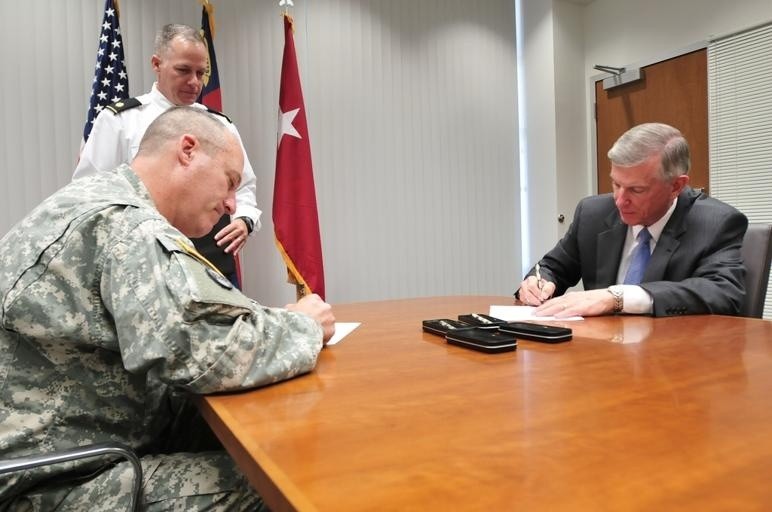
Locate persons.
[513,122,748,319]
[71,22,265,291]
[0,104,337,511]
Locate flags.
[268,12,327,303]
[79,0,132,168]
[194,3,245,294]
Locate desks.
[186,293,772,512]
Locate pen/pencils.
[297,283,305,303]
[536,263,542,290]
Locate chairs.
[0,440,143,512]
[740,221,772,319]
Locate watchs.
[235,215,255,237]
[606,284,624,313]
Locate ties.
[623,227,652,285]
